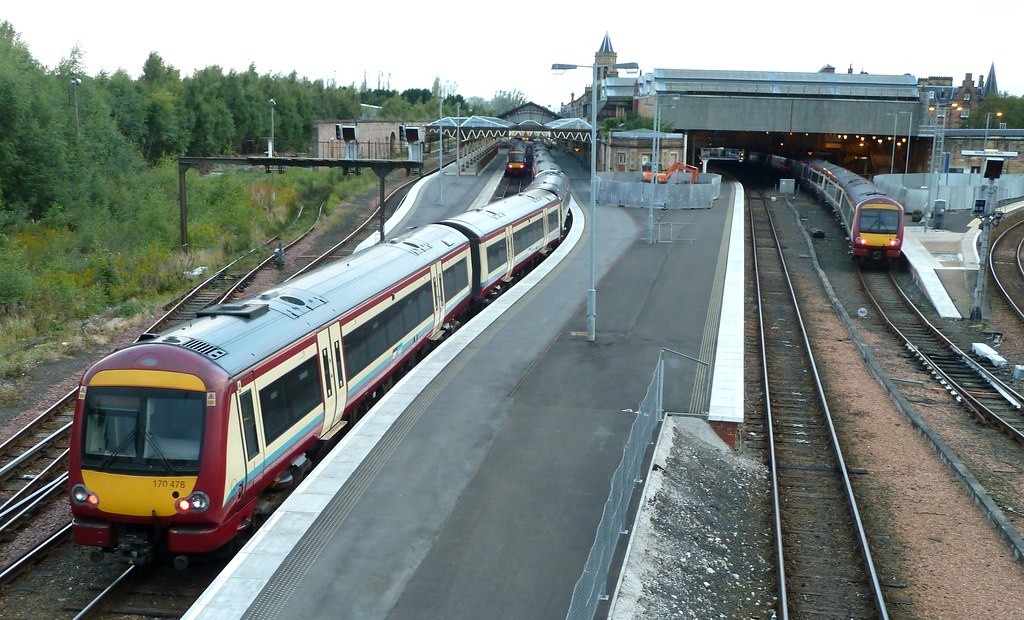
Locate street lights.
[980,111,1002,184]
[643,100,679,212]
[923,102,964,232]
[550,60,642,341]
[634,85,685,245]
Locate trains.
[505,141,528,178]
[739,148,906,273]
[67,140,571,571]
